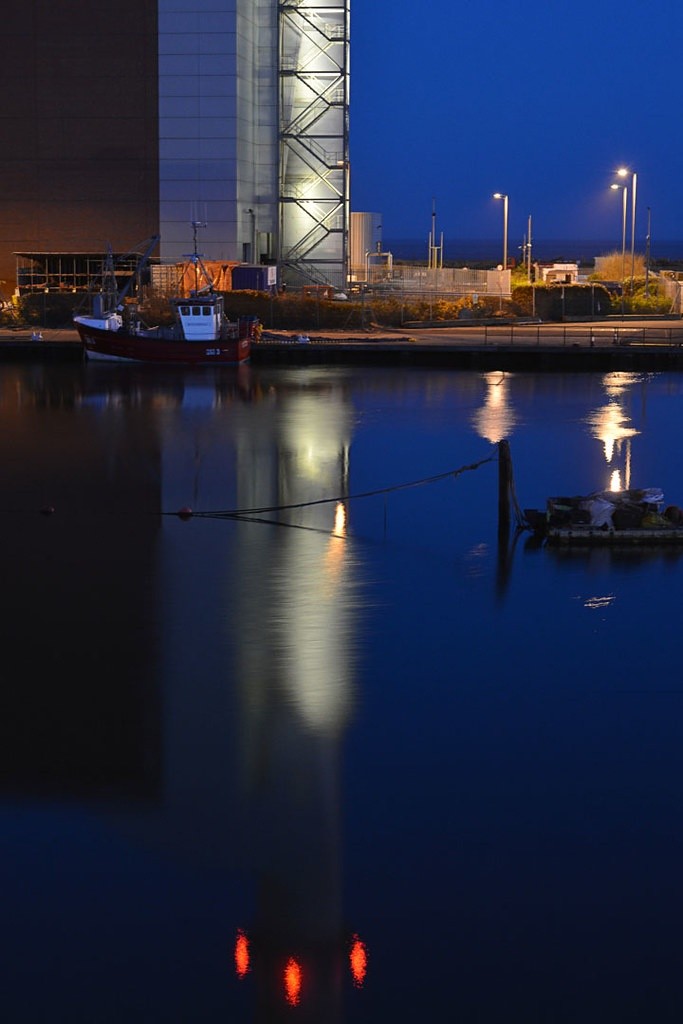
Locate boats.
[522,485,683,548]
[71,199,264,364]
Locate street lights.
[610,168,638,300]
[492,193,509,270]
